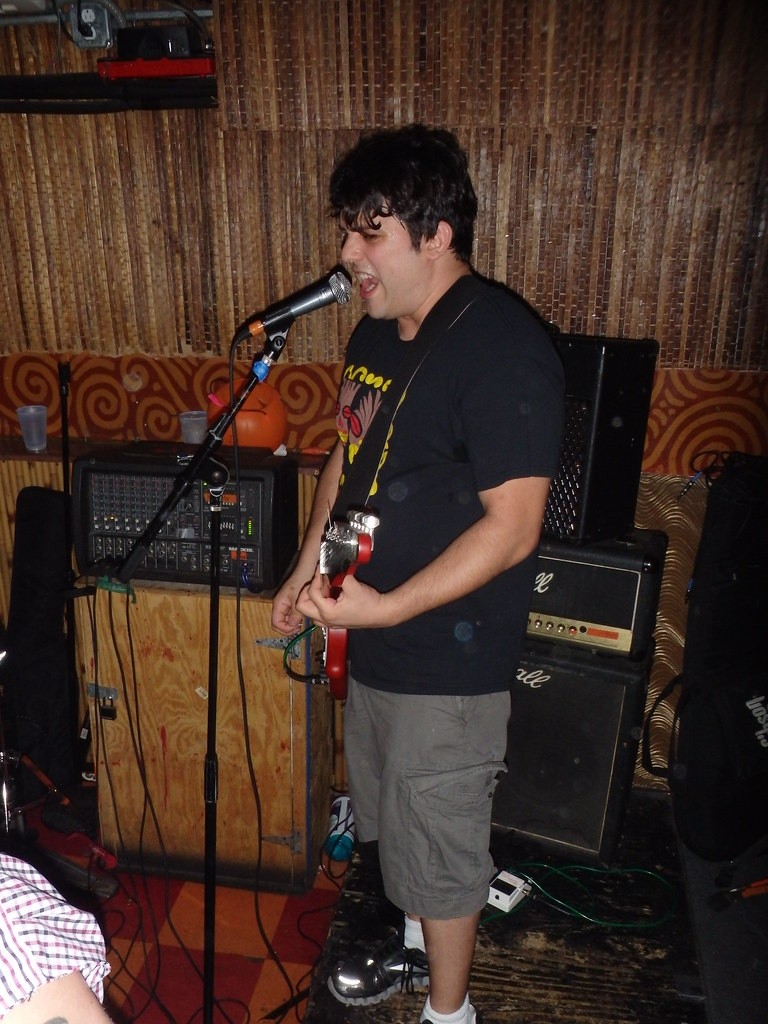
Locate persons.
[0,854,111,1024]
[271,121,566,1024]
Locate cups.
[179,410,208,443]
[16,405,48,452]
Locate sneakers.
[326,937,482,1024]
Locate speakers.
[669,450,768,895]
[485,653,656,873]
[538,335,658,545]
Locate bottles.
[326,796,356,861]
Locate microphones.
[237,271,352,341]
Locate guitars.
[321,507,378,698]
